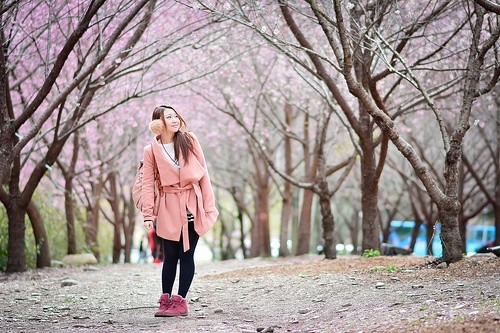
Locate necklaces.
[160,138,180,169]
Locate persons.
[142,105,215,318]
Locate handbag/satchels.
[133,160,159,216]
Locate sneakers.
[164,295,188,317]
[154,293,170,316]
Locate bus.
[383,217,496,259]
